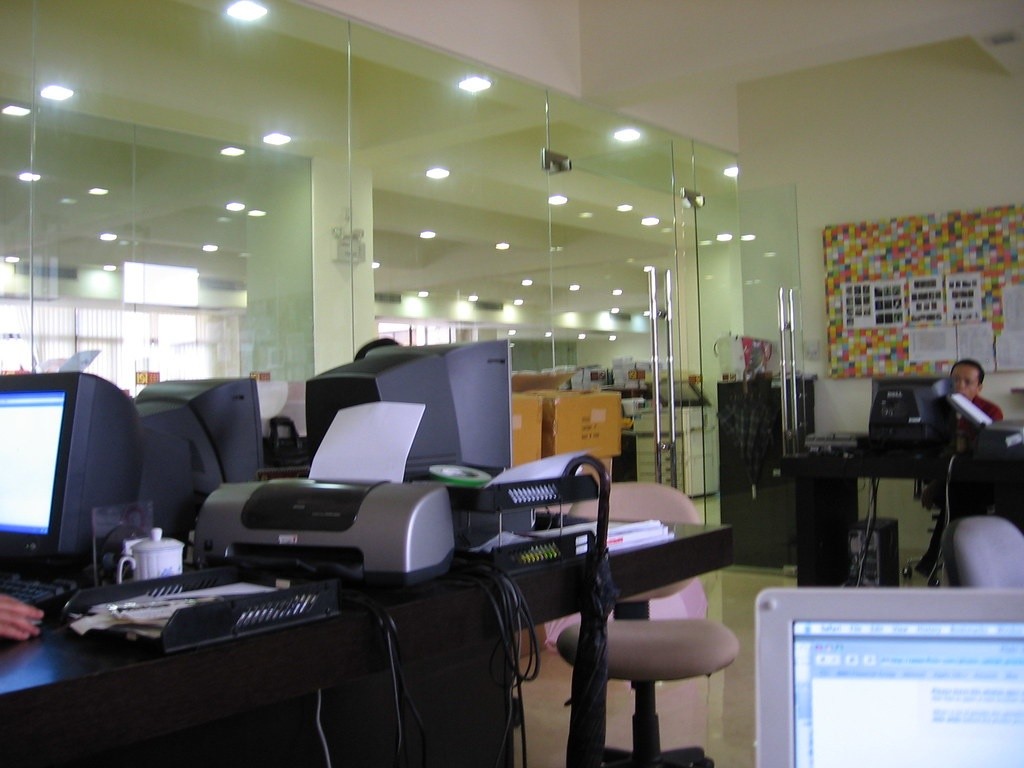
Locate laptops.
[754,587,1024,768]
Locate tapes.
[428,464,491,487]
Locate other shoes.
[915,555,938,577]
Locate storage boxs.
[510,368,628,467]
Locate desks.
[0,516,736,768]
[778,457,1024,586]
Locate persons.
[914,359,1004,579]
[0,594,44,640]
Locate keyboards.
[534,513,591,530]
[0,574,76,611]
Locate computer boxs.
[848,518,899,586]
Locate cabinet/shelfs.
[634,404,720,498]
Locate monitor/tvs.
[1,339,513,579]
[868,374,954,452]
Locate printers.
[193,478,456,591]
[945,394,1024,464]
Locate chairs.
[554,482,740,768]
[945,515,1024,589]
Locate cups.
[116,527,185,584]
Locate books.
[519,520,674,555]
